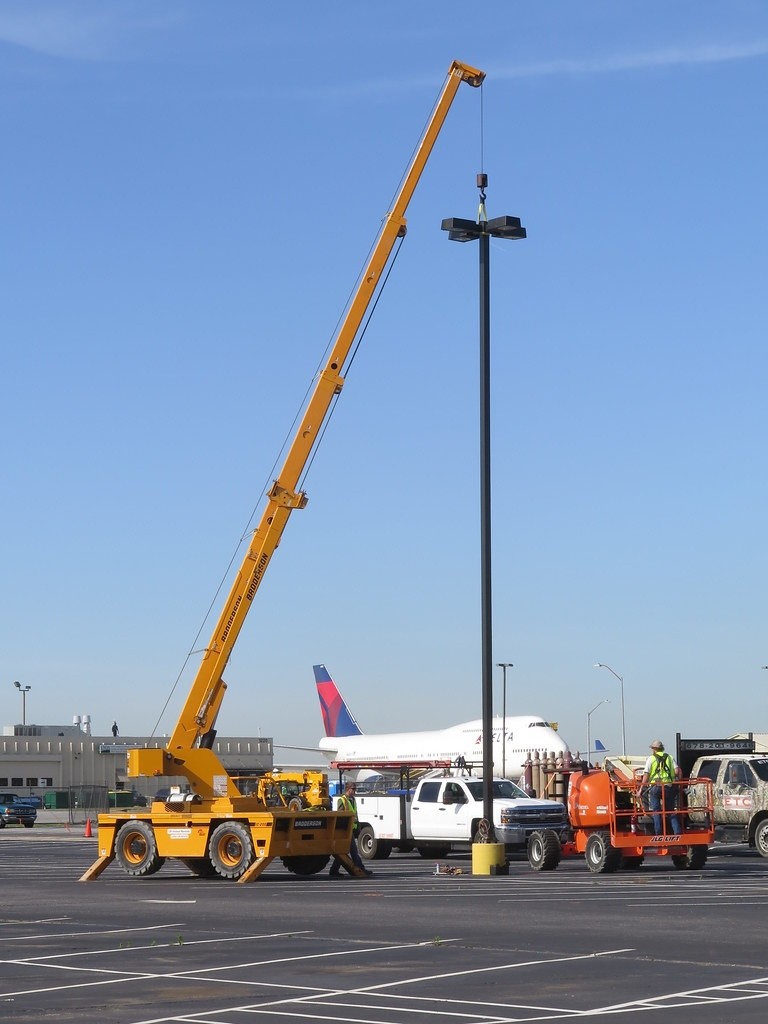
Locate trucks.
[603,732,768,858]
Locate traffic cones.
[83,818,93,837]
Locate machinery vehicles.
[526,760,716,874]
[76,55,490,882]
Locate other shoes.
[367,871,372,874]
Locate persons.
[638,742,682,850]
[329,782,372,877]
[111,721,119,737]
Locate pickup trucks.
[325,775,569,860]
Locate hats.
[345,782,356,789]
[649,740,663,749]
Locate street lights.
[595,663,626,755]
[440,213,527,876]
[496,663,514,778]
[14,681,32,724]
[586,700,609,768]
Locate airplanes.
[272,663,573,789]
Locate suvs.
[0,794,38,829]
